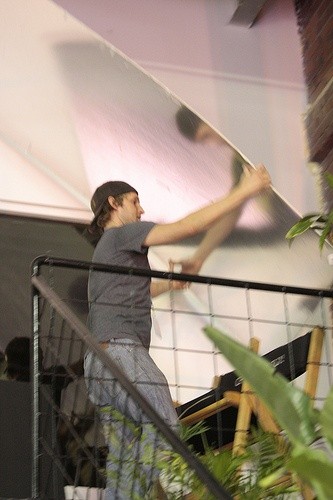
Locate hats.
[88,181,139,232]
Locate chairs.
[155,326,326,500]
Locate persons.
[83,162,271,500]
[5,335,107,500]
[166,104,306,279]
